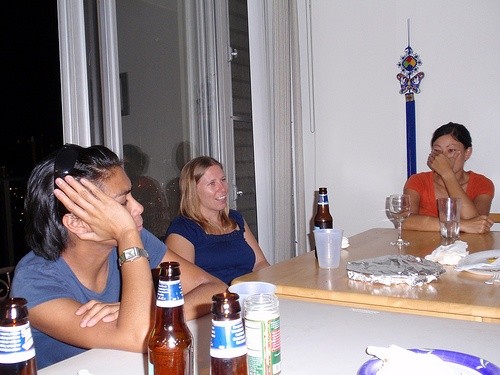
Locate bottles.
[314,187,334,257]
[0,297,39,374]
[210,293,247,375]
[148,261,195,375]
[244,293,282,375]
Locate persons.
[163,156,270,287]
[397,122,494,235]
[7,143,230,373]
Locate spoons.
[455,257,500,272]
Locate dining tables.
[229,222,500,319]
[33,293,500,375]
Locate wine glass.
[389,194,411,246]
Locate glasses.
[52,142,75,207]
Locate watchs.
[117,246,149,268]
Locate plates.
[358,349,500,375]
[460,250,500,276]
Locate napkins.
[424,239,469,265]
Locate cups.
[438,197,462,242]
[314,229,344,268]
[229,282,275,319]
